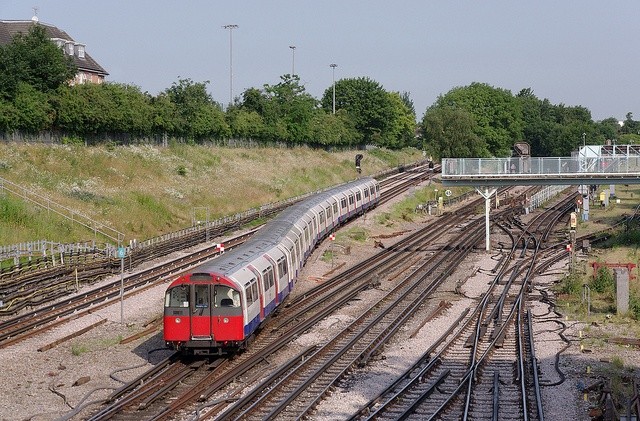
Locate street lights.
[288,43,299,93]
[329,62,339,116]
[223,22,238,113]
